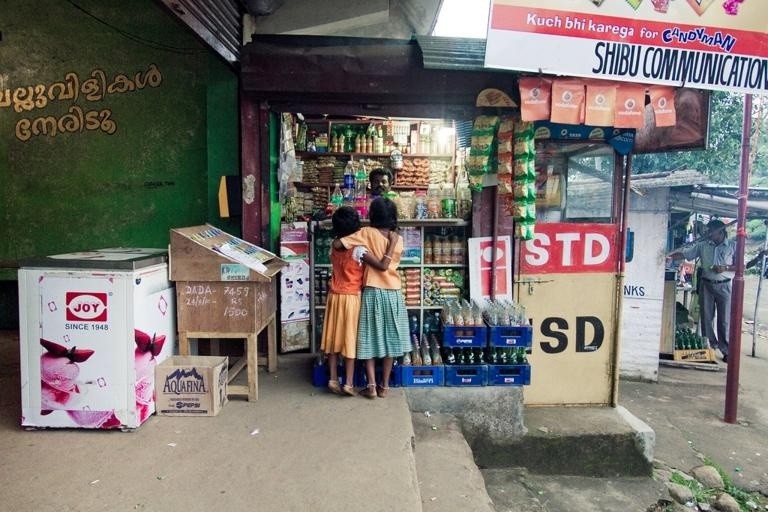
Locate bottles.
[423,234,464,264]
[675,327,712,363]
[419,131,454,156]
[404,296,528,377]
[308,123,384,154]
[329,159,367,221]
[387,180,472,221]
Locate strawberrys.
[41,337,94,363]
[136,330,166,356]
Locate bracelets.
[384,255,392,260]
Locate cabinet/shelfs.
[309,218,472,354]
[293,116,456,217]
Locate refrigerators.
[16,242,176,430]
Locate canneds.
[315,273,328,306]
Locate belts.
[702,276,731,286]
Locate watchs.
[726,265,729,271]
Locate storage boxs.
[155,355,229,416]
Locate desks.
[170,223,290,401]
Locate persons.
[369,168,409,219]
[334,196,414,399]
[669,220,736,363]
[320,206,399,396]
